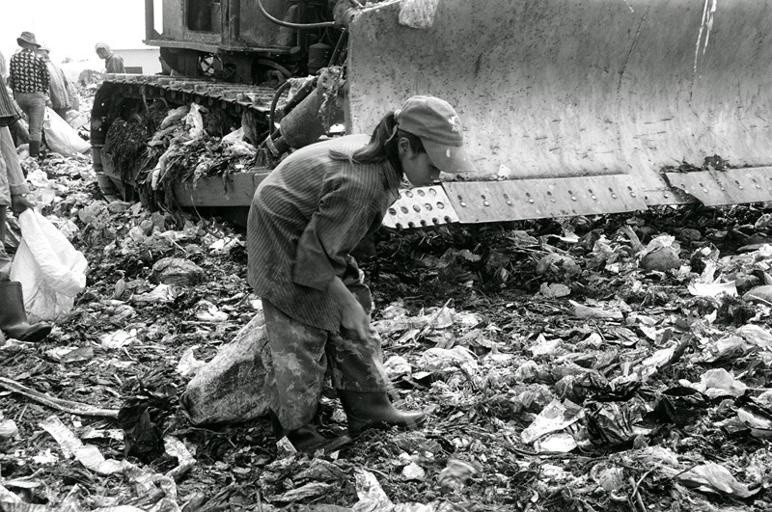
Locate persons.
[1,241,52,342]
[0,74,36,244]
[95,42,127,76]
[244,93,472,451]
[9,30,52,160]
[38,46,72,121]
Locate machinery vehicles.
[89,0,772,231]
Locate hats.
[16,31,41,49]
[397,94,479,174]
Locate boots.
[0,281,53,342]
[337,387,429,437]
[289,422,354,457]
